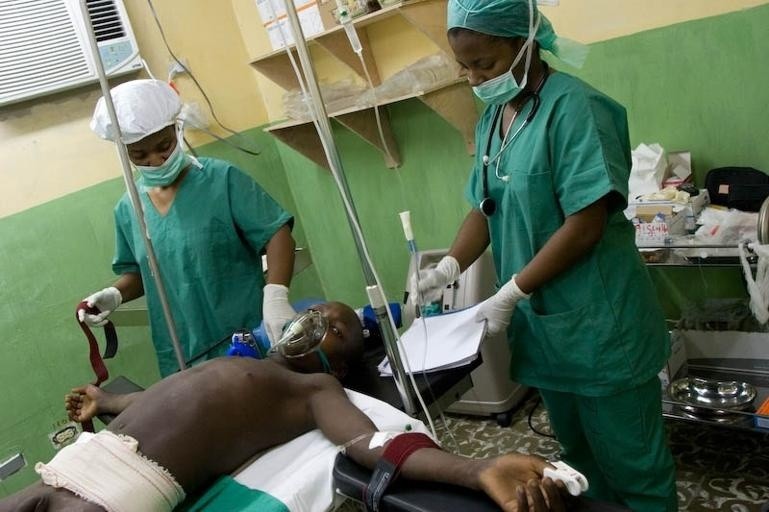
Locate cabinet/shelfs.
[249,0,468,134]
[634,196,769,438]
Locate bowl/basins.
[667,376,757,417]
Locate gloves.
[410,256,460,307]
[263,283,299,352]
[475,273,534,341]
[78,287,123,326]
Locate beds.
[176,322,484,512]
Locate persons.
[78,79,297,377]
[408,1,680,511]
[0,301,577,512]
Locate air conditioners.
[0,0,144,111]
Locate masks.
[468,40,533,107]
[126,126,191,188]
